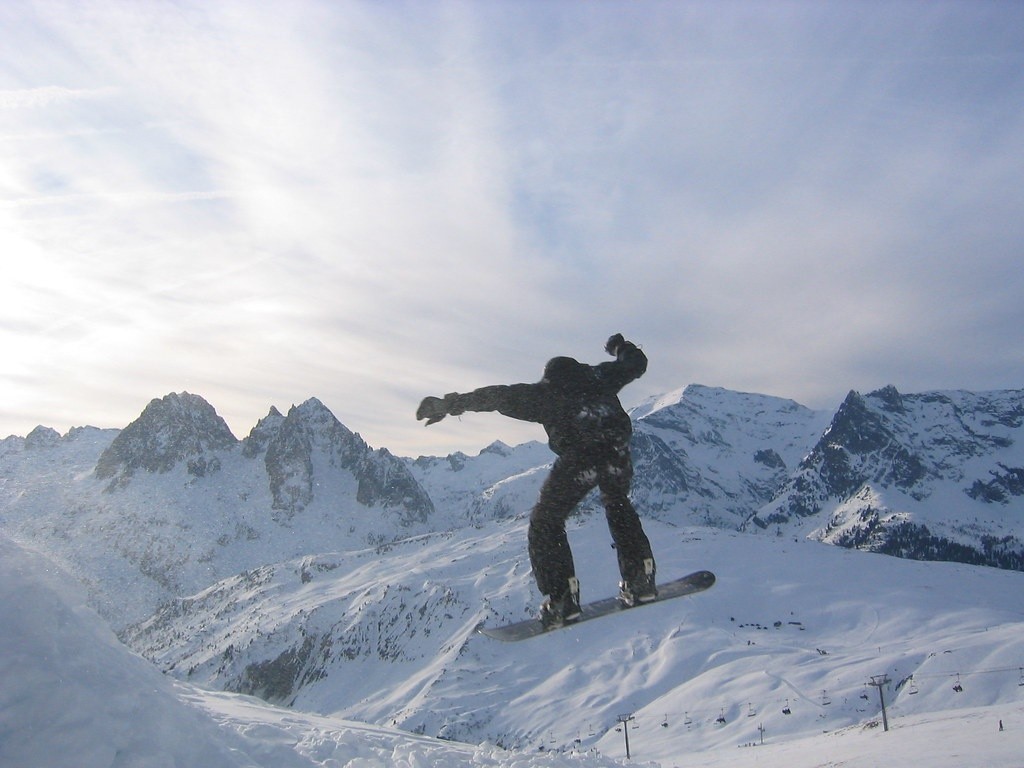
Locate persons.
[415,333,660,625]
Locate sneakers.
[618,558,658,610]
[540,577,580,620]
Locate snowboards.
[478,570,716,643]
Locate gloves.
[416,396,447,426]
[606,333,625,356]
